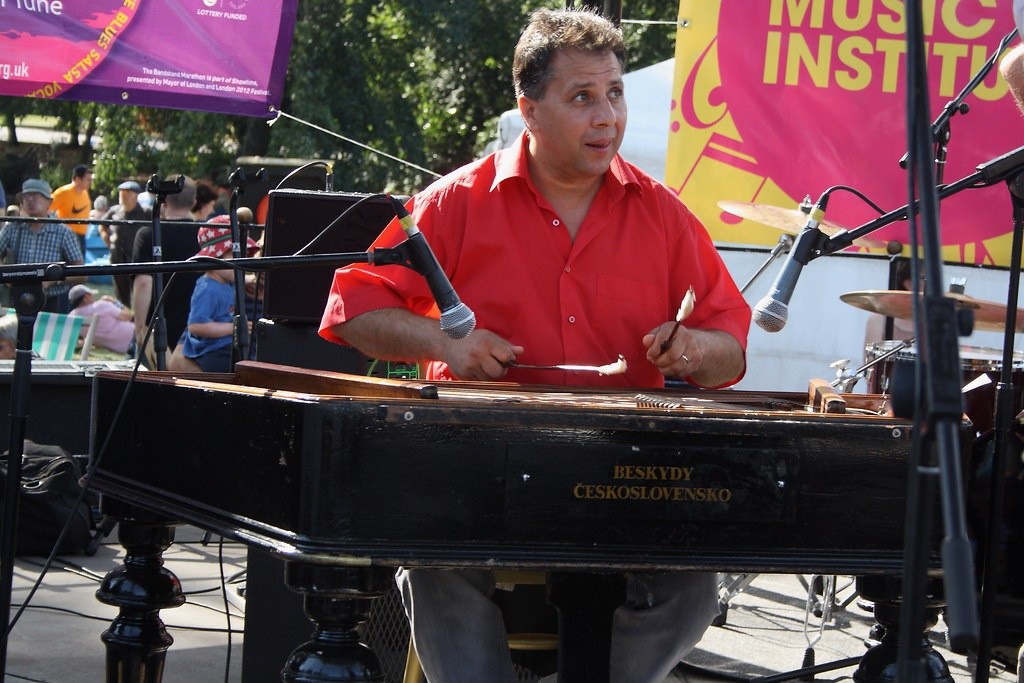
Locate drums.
[862,342,1024,473]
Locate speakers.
[265,188,412,324]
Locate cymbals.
[715,201,887,249]
[840,291,1024,334]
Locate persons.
[320,7,755,683]
[863,257,925,396]
[0,165,264,374]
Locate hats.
[185,215,260,263]
[17,179,54,200]
[117,181,142,194]
[68,284,100,299]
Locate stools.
[403,569,560,683]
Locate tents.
[497,56,1024,395]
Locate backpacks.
[1,439,95,556]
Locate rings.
[681,354,689,363]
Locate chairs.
[0,307,100,362]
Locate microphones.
[389,201,477,339]
[752,192,829,334]
